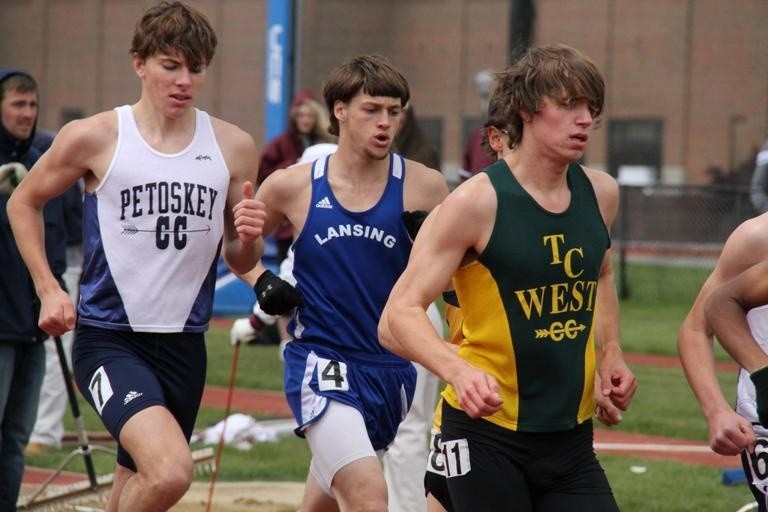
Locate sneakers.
[22,444,56,457]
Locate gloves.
[227,317,257,346]
[252,270,302,318]
[750,363,768,433]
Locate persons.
[241,49,448,512]
[676,212,768,512]
[0,70,87,511]
[5,4,268,512]
[378,46,635,511]
